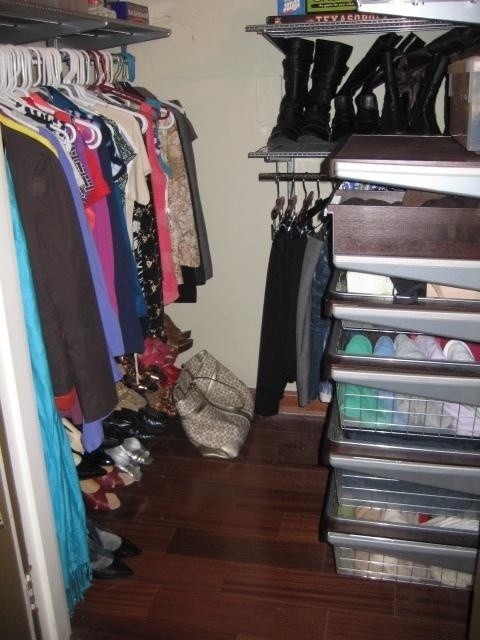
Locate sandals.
[115,382,146,412]
[86,524,141,557]
[79,479,121,511]
[71,452,108,480]
[120,437,154,466]
[88,552,134,580]
[108,449,142,482]
[62,416,113,465]
[98,465,134,489]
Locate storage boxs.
[448,55,480,155]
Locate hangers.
[258,157,336,242]
[1,45,185,154]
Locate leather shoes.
[134,409,167,427]
[126,374,158,392]
[108,420,154,442]
[143,367,164,382]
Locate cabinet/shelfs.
[244,19,480,591]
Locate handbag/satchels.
[172,349,256,460]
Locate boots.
[266,37,314,151]
[296,38,354,146]
[329,32,403,146]
[410,52,449,136]
[381,50,404,136]
[355,32,425,135]
